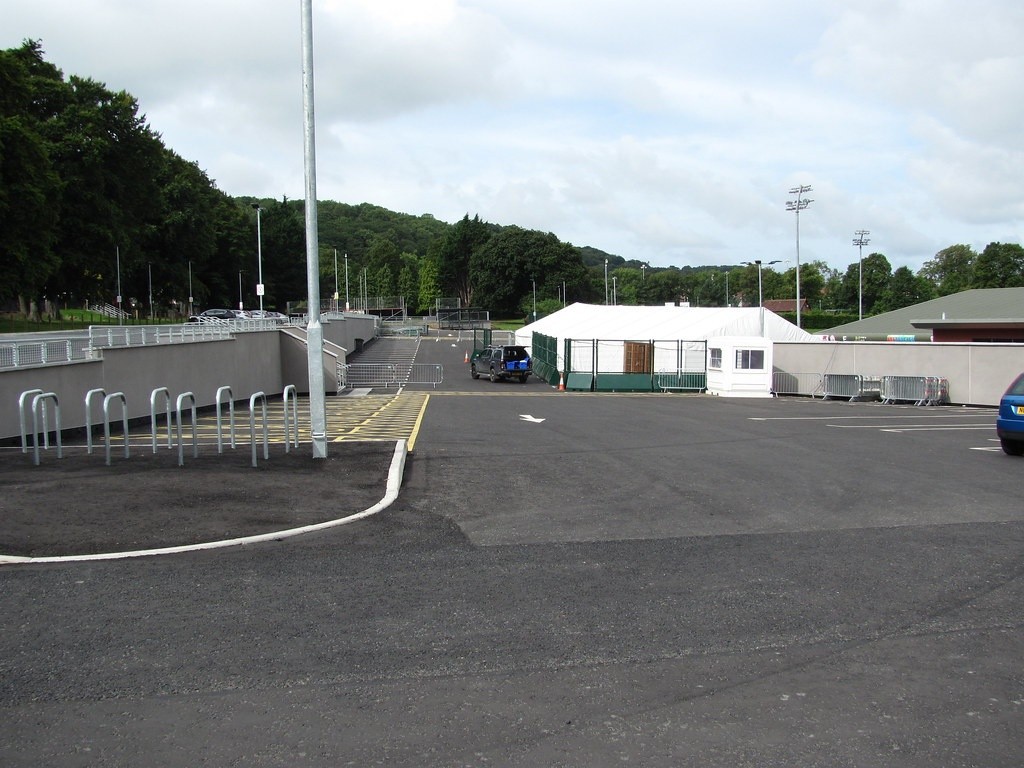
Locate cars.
[188,307,306,326]
[996,372,1024,456]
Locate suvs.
[469,345,533,384]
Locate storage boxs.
[519,357,529,369]
[506,361,517,370]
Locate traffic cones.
[464,350,470,363]
[556,374,566,392]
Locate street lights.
[529,278,536,322]
[783,184,814,328]
[557,285,561,302]
[640,264,648,280]
[852,230,873,321]
[604,259,609,305]
[238,269,248,311]
[188,260,196,317]
[147,261,154,318]
[331,244,340,312]
[724,271,730,307]
[249,202,268,319]
[611,276,618,305]
[561,278,566,308]
[739,260,782,307]
